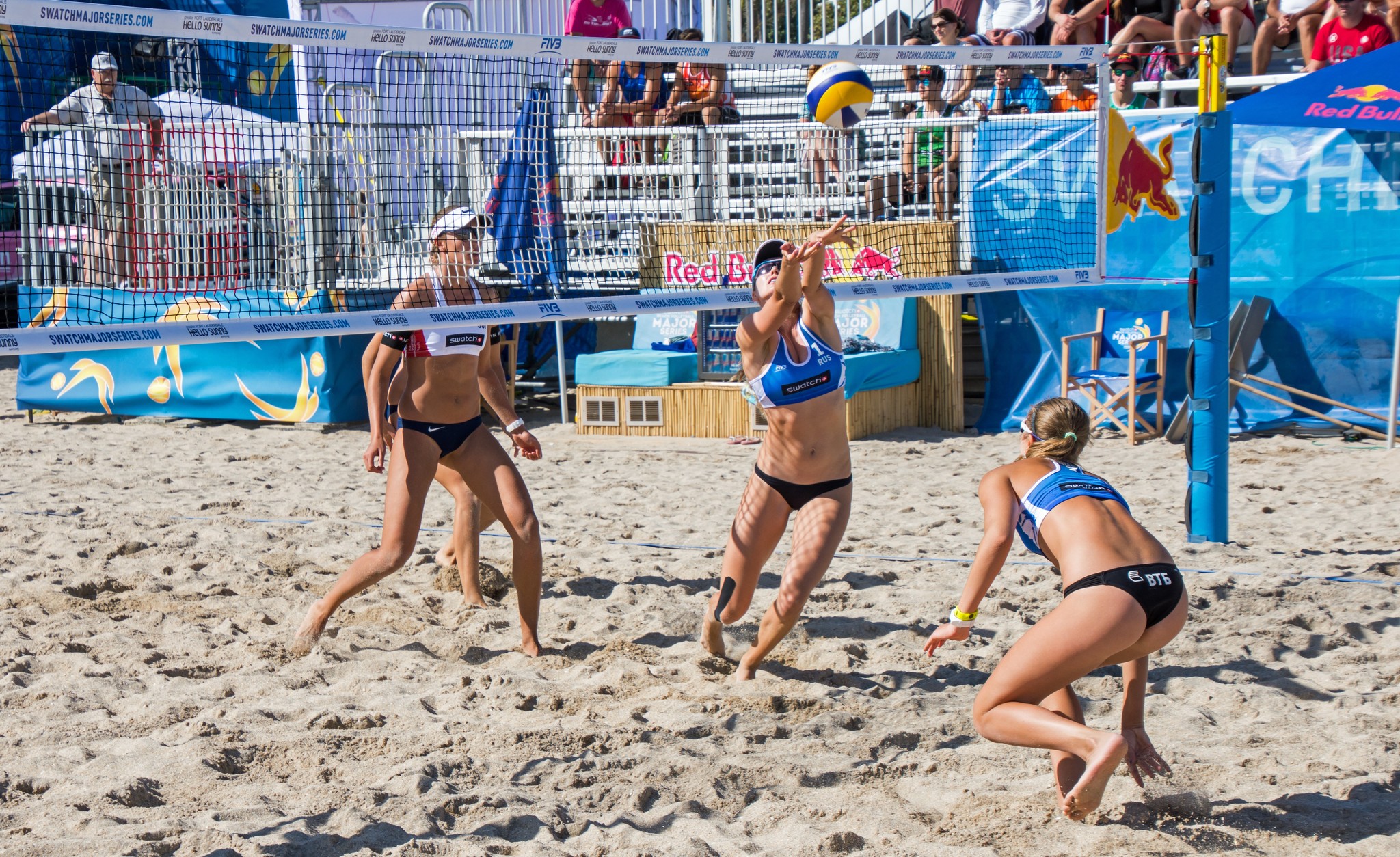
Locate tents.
[10,90,344,186]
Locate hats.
[910,65,946,83]
[91,51,118,72]
[617,28,640,39]
[753,238,797,276]
[425,206,492,251]
[1051,63,1088,72]
[1109,52,1139,71]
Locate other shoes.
[814,206,832,221]
[1035,75,1051,86]
[636,176,660,189]
[1165,64,1188,81]
[1083,71,1096,79]
[900,101,919,117]
[1226,62,1234,77]
[837,181,854,197]
[597,178,615,189]
[660,175,676,189]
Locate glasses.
[441,227,486,240]
[1112,69,1139,77]
[752,259,792,289]
[102,97,114,113]
[930,20,952,32]
[1056,68,1085,75]
[1020,420,1046,443]
[995,63,1015,68]
[916,79,935,86]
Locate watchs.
[950,610,975,629]
[1205,0,1211,11]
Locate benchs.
[559,61,1315,290]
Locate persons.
[21,51,165,289]
[336,0,1400,281]
[925,397,1191,822]
[700,215,856,677]
[290,204,544,659]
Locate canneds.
[708,308,745,373]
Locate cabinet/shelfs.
[697,307,762,380]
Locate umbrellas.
[1183,42,1400,132]
[479,81,569,422]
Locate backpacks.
[960,96,989,131]
[1140,45,1177,98]
[612,141,643,188]
[911,14,944,43]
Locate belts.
[92,158,142,168]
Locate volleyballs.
[807,61,874,128]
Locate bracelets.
[955,605,978,620]
[505,418,524,433]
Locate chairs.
[575,289,699,386]
[480,323,520,425]
[835,297,922,401]
[1060,308,1170,448]
